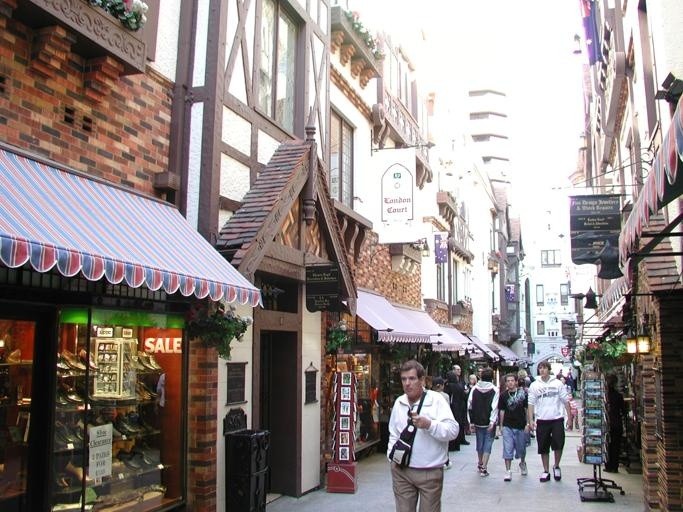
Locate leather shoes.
[65,461,92,484]
[116,450,141,471]
[131,350,162,371]
[68,424,84,441]
[113,412,154,435]
[60,349,87,372]
[58,384,84,404]
[55,421,75,443]
[136,382,159,401]
[96,416,122,437]
[55,393,69,407]
[78,348,100,371]
[55,469,68,487]
[129,446,153,467]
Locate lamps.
[626,327,637,355]
[598,241,624,280]
[638,323,654,355]
[585,287,602,309]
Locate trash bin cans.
[225,429,272,512]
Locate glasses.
[408,409,414,424]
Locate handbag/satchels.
[390,420,416,467]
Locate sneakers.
[520,462,528,475]
[443,462,452,470]
[553,465,561,480]
[477,462,483,471]
[504,471,512,480]
[480,468,489,477]
[540,472,550,482]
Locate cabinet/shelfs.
[93,338,137,397]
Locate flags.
[434,233,448,263]
[505,287,515,302]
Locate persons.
[157,374,165,412]
[387,361,460,512]
[425,360,584,482]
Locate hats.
[432,377,445,386]
